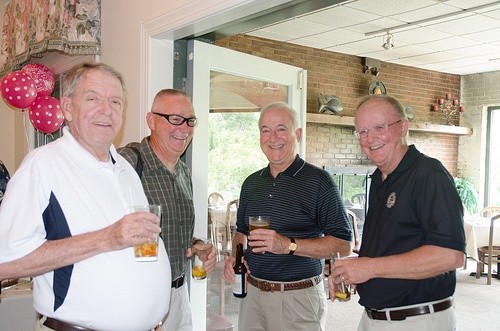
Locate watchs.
[288,237,297,255]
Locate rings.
[340,274,345,280]
[263,241,264,247]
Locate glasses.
[150,112,198,127]
[353,120,403,140]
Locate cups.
[328,251,353,302]
[134,205,162,262]
[248,215,271,255]
[191,238,213,281]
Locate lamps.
[365,58,381,76]
[381,31,396,51]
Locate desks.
[210,206,238,231]
[464,215,500,269]
[346,203,366,218]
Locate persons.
[117,89,218,331]
[221,102,353,331]
[328,94,467,331]
[0,59,172,331]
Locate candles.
[438,98,444,105]
[433,105,438,109]
[459,106,464,112]
[446,93,452,100]
[453,100,459,104]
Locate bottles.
[233,243,247,298]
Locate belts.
[171,273,185,289]
[246,276,323,293]
[364,300,452,321]
[38,314,164,331]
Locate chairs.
[351,193,366,205]
[346,209,360,254]
[476,206,500,285]
[208,192,239,315]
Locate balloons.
[1,63,64,135]
